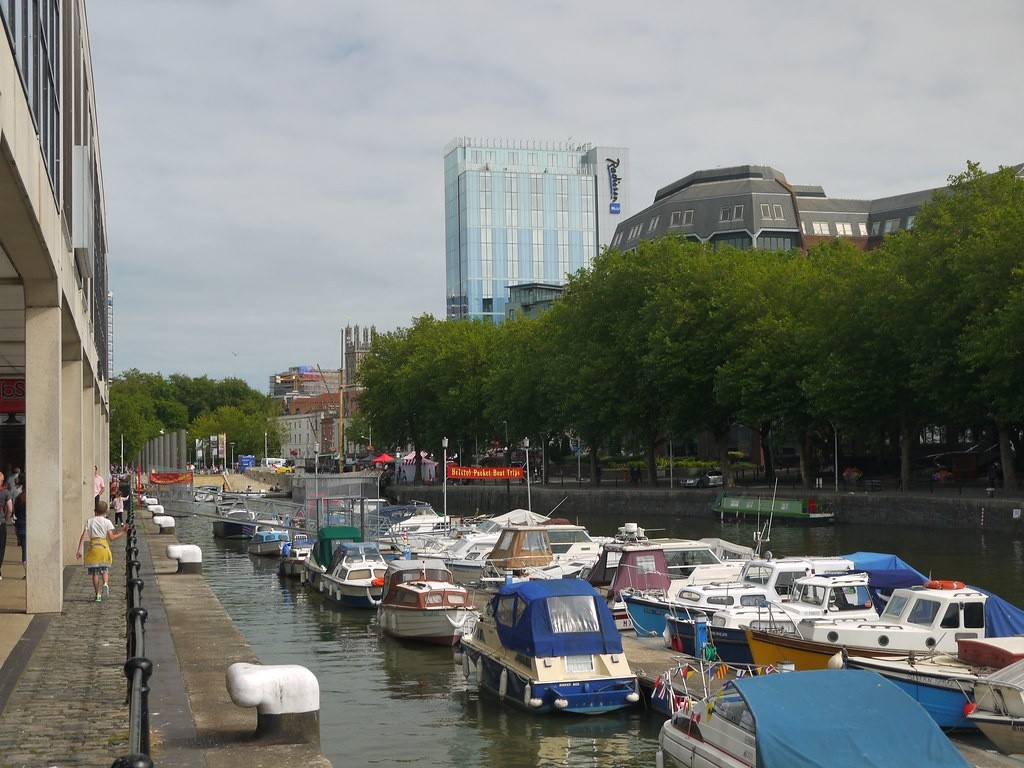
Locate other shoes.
[95,594,102,601]
[102,584,109,601]
[121,523,123,526]
[115,525,119,527]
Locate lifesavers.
[923,580,964,589]
[371,578,384,586]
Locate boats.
[193,483,1024,763]
[656,658,973,768]
[706,480,838,526]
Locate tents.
[361,453,394,462]
[400,451,435,484]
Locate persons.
[76,501,128,602]
[109,464,121,509]
[113,491,129,527]
[402,470,408,484]
[0,468,26,580]
[94,466,105,504]
[709,464,716,471]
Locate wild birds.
[159,428,165,435]
[827,644,848,669]
[232,352,240,356]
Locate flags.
[655,662,775,724]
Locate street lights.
[503,420,508,444]
[361,435,372,448]
[313,440,322,500]
[456,440,465,485]
[441,436,448,538]
[524,436,531,512]
[230,442,236,475]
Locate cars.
[913,432,1023,488]
[680,467,723,488]
